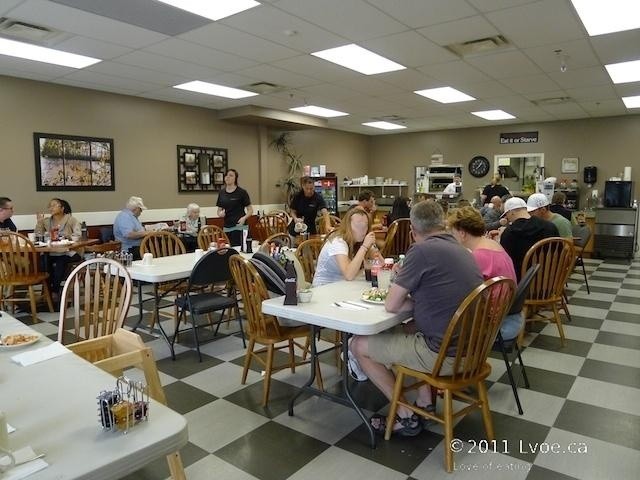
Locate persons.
[497,194,564,297]
[444,206,526,344]
[33,198,85,293]
[308,205,384,382]
[171,202,205,235]
[283,163,582,260]
[108,194,162,261]
[348,199,487,436]
[215,168,254,248]
[0,195,34,313]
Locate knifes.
[342,300,371,311]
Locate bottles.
[80,219,89,241]
[382,258,394,270]
[198,217,201,233]
[395,254,407,266]
[44,232,50,243]
[285,261,299,304]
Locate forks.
[334,303,358,311]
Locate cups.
[296,290,313,303]
[71,233,80,241]
[27,232,37,242]
[364,259,379,281]
[294,223,307,233]
[376,268,391,292]
[371,265,382,288]
[195,249,204,259]
[141,253,154,266]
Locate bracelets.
[360,243,371,252]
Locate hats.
[128,196,148,209]
[527,194,550,213]
[499,197,527,219]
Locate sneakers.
[341,350,369,382]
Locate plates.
[0,330,42,349]
[360,297,386,305]
[369,176,409,186]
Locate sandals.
[370,412,422,437]
[412,401,439,427]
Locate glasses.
[2,207,12,210]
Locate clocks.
[467,156,490,178]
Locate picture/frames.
[176,144,229,193]
[32,128,117,193]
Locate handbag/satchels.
[248,233,297,297]
